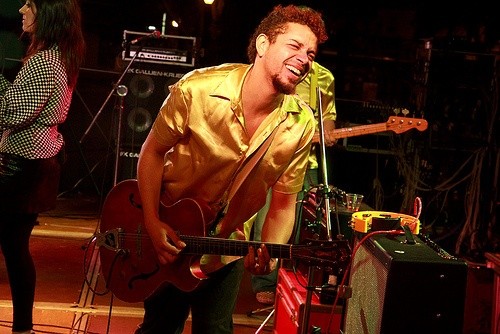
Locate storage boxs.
[272,268,343,334]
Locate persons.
[1,0,85,334]
[136,5,330,334]
[252,60,337,306]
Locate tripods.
[247,303,277,334]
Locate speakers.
[115,62,195,184]
[339,231,468,334]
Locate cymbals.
[348,209,423,235]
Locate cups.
[346,194,363,211]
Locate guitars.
[313,109,429,146]
[99,178,352,303]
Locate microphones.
[306,284,352,298]
[131,31,160,44]
